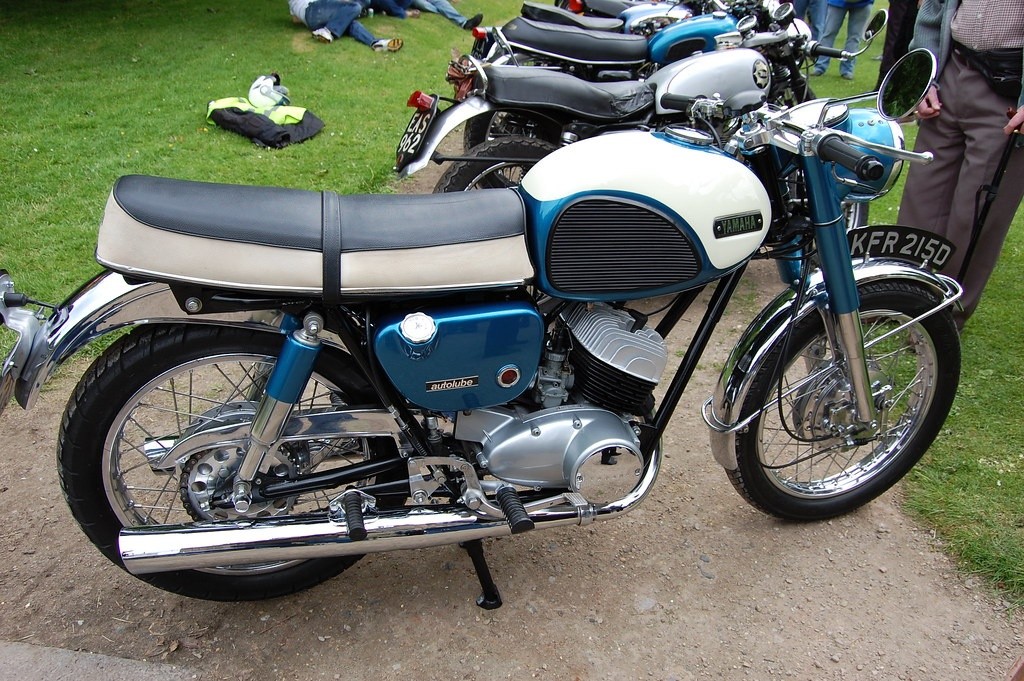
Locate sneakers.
[312,28,332,44]
[372,38,404,52]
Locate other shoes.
[462,13,483,30]
[811,72,821,76]
[841,74,853,79]
[409,9,420,17]
[872,55,882,60]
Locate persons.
[873,0,923,95]
[857,0,1023,352]
[779,0,820,41]
[287,0,483,52]
[806,0,874,80]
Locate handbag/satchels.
[952,42,1023,98]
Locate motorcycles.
[392,0,888,283]
[0,46,966,612]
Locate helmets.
[248,72,291,108]
